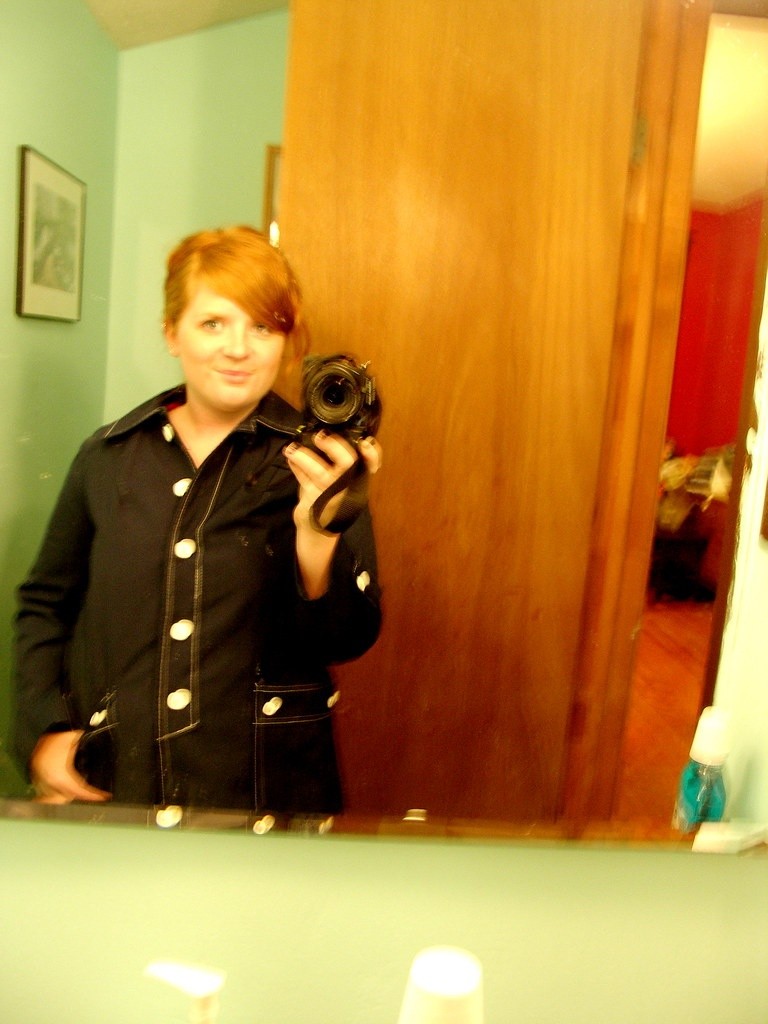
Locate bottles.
[670,706,732,831]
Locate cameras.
[302,353,385,465]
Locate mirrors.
[2,0,768,839]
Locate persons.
[14,224,384,817]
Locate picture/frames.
[16,145,85,322]
